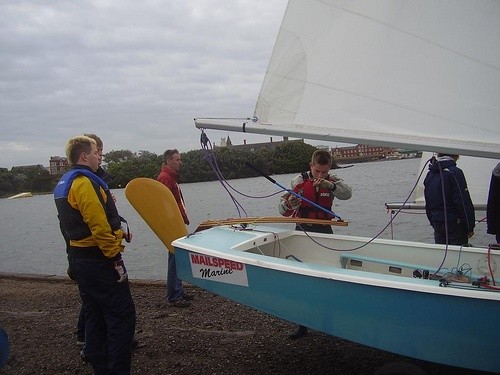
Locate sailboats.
[384,151,500,214]
[123,0,500,375]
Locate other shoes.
[171,299,190,307]
[290,327,306,339]
[79,348,97,365]
[76,337,86,345]
[181,292,195,300]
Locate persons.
[423,154,475,246]
[53,135,136,375]
[278,151,352,339]
[485,162,500,245]
[157,149,194,307]
